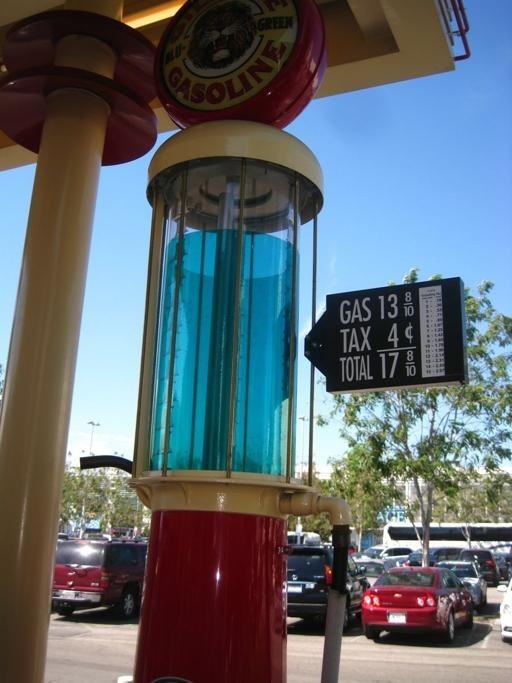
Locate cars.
[50,533,148,619]
[288,548,512,641]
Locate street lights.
[295,415,309,544]
[78,419,102,538]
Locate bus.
[287,531,320,547]
[383,522,511,554]
